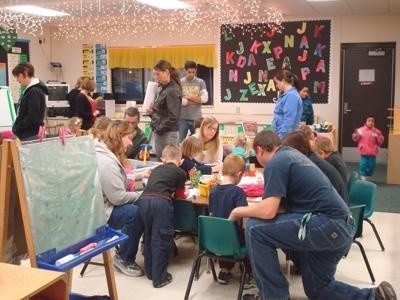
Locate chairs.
[184,220,258,299]
[346,171,386,286]
[166,199,219,274]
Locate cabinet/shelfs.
[218,122,337,162]
[111,118,157,157]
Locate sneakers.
[114,252,172,287]
[218,271,233,284]
[375,281,397,300]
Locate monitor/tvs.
[44,83,71,108]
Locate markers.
[79,243,97,254]
[55,253,78,267]
[107,235,119,244]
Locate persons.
[208,154,257,288]
[282,124,349,204]
[67,76,90,116]
[228,132,397,300]
[232,133,256,164]
[145,59,182,161]
[352,116,384,181]
[63,106,223,288]
[179,60,208,142]
[298,84,314,124]
[271,70,304,140]
[12,62,49,142]
[74,78,103,127]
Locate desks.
[117,169,293,290]
[0,258,70,300]
